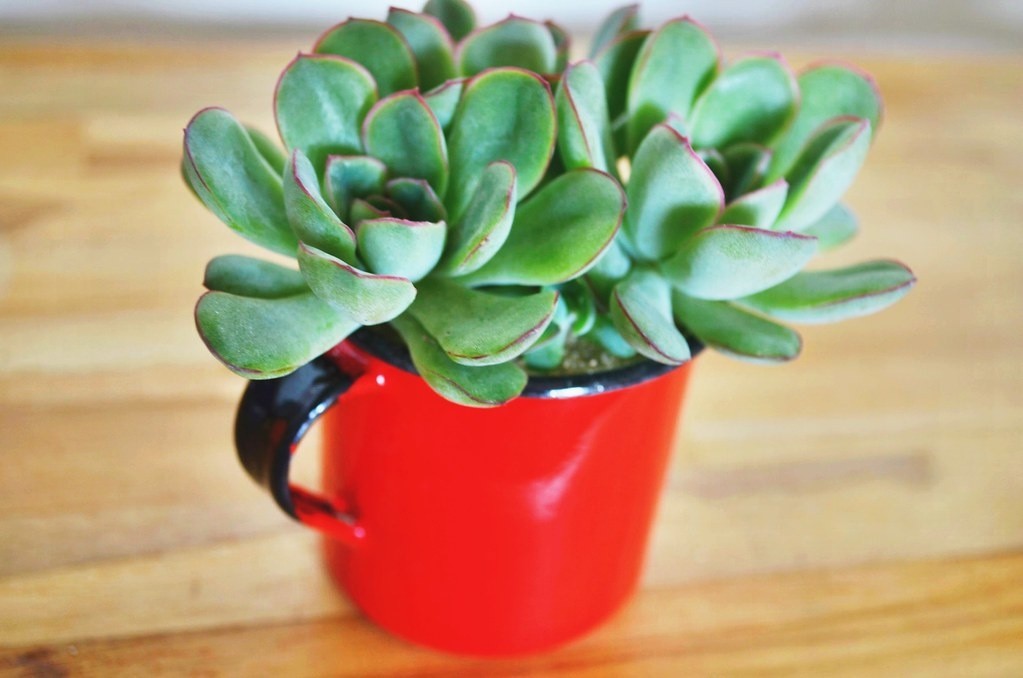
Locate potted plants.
[176,0,919,657]
